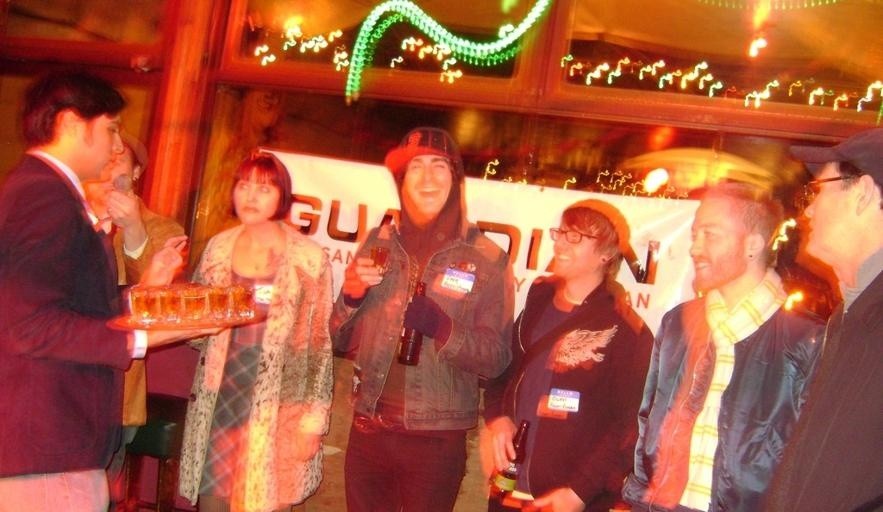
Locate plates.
[104,312,267,330]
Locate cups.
[145,286,159,322]
[159,284,182,327]
[230,278,255,320]
[129,285,148,325]
[182,282,207,320]
[207,282,231,320]
[369,246,392,276]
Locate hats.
[788,128,883,184]
[568,199,629,253]
[385,127,456,173]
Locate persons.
[330,127,514,512]
[0,66,228,511]
[178,151,333,512]
[620,182,825,511]
[764,127,883,511]
[83,127,186,512]
[483,201,655,512]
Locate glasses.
[549,228,608,243]
[784,172,856,207]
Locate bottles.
[486,420,532,511]
[397,283,426,365]
